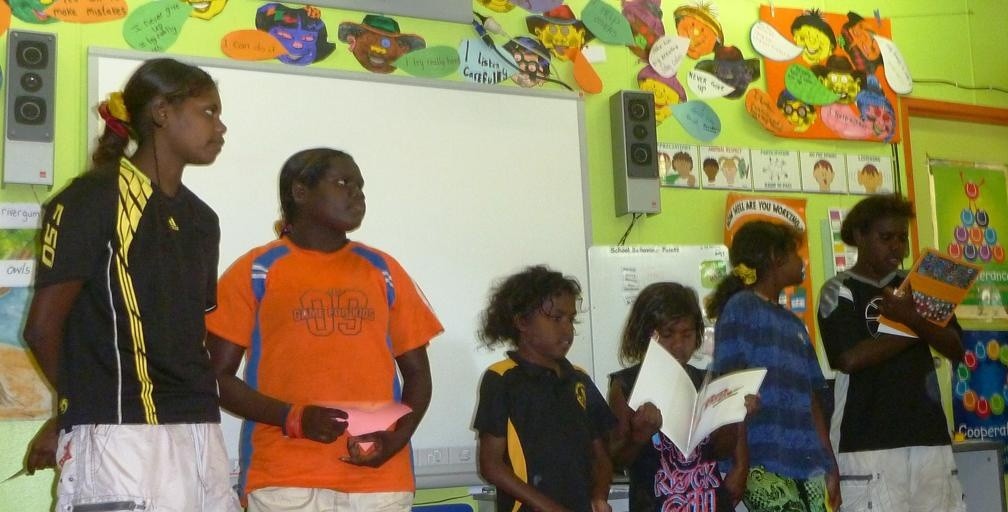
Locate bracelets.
[280,401,304,440]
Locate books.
[877,245,981,342]
[628,337,769,459]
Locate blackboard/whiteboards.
[85,47,594,491]
[952,441,1007,512]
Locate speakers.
[0,26,58,187]
[610,88,663,215]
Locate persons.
[471,266,618,510]
[705,221,843,511]
[813,195,970,511]
[604,280,759,512]
[200,148,445,512]
[20,59,245,510]
